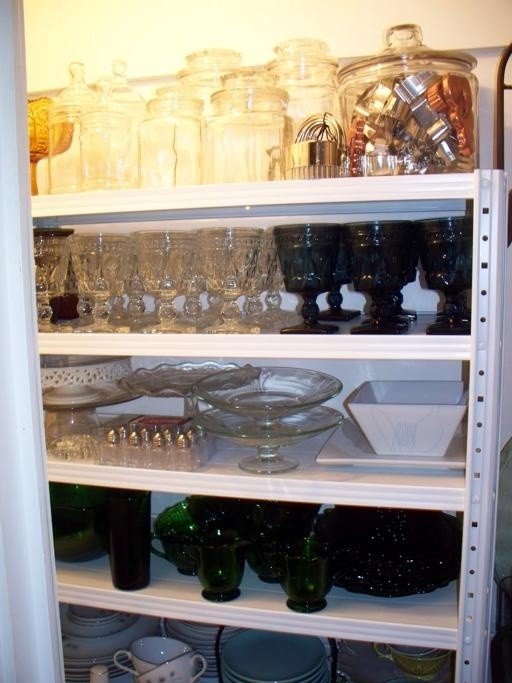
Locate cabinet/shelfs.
[31,165,511,681]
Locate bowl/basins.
[347,377,468,458]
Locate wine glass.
[194,404,345,474]
[34,215,472,334]
[121,362,260,415]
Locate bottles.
[29,25,486,193]
[90,419,216,471]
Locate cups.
[370,642,453,682]
[47,481,332,611]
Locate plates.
[198,367,340,421]
[58,600,358,682]
[317,420,467,472]
